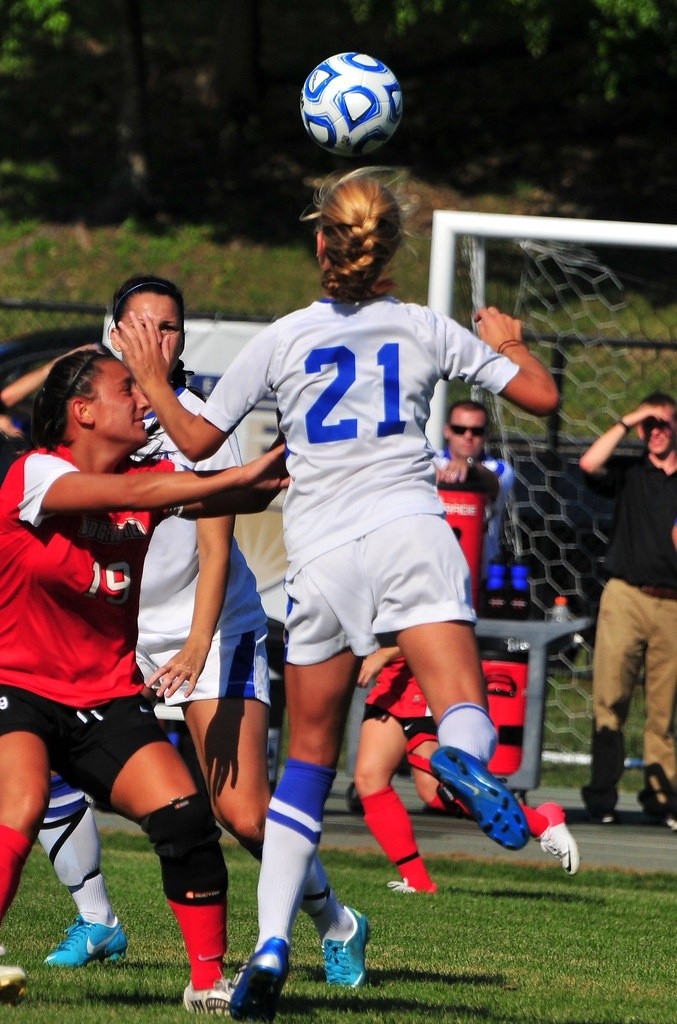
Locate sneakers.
[387,878,438,894]
[184,967,236,1017]
[44,916,128,969]
[229,935,291,1023]
[533,802,579,875]
[320,905,371,988]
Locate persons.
[37,274,372,990]
[0,348,290,1017]
[580,394,677,833]
[113,168,559,1024]
[0,344,106,455]
[430,400,514,579]
[354,644,580,894]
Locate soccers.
[299,51,404,158]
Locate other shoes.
[0,964,25,1008]
[429,745,529,851]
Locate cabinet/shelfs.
[334,614,593,812]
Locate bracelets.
[498,340,529,354]
[619,420,630,431]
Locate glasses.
[446,423,488,438]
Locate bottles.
[485,553,527,591]
[551,596,574,624]
